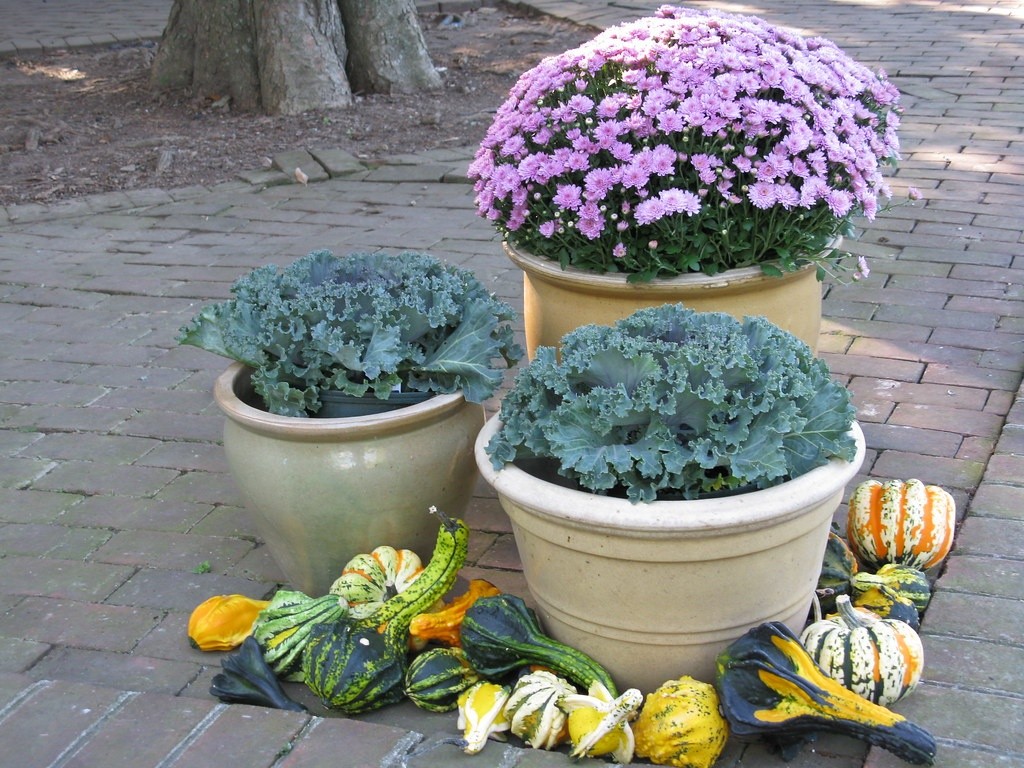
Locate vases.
[215,361,486,598]
[503,231,844,368]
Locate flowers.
[466,6,922,286]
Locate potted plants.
[474,302,868,705]
[173,252,524,419]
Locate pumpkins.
[187,479,956,768]
